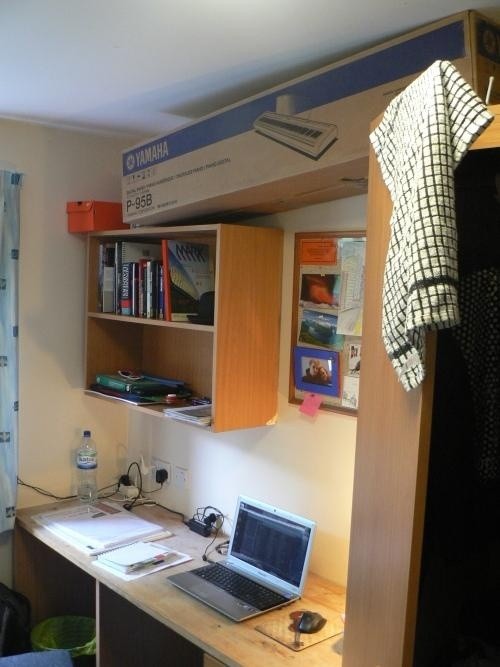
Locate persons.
[317,366,332,386]
[302,358,325,385]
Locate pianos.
[253,110,338,161]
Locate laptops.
[167,495,316,623]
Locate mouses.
[299,610,326,633]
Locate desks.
[12,489,344,665]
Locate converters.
[156,470,167,482]
[188,519,211,536]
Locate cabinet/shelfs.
[83,231,220,430]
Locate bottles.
[76,430,97,503]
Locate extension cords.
[119,484,139,498]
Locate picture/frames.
[287,231,368,419]
[294,346,341,398]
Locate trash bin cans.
[29,615,97,666]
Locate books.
[96,539,171,577]
[95,371,163,393]
[91,541,193,582]
[96,240,214,326]
[163,400,213,427]
[31,499,174,558]
[85,383,188,408]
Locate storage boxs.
[122,10,500,228]
[65,201,129,234]
